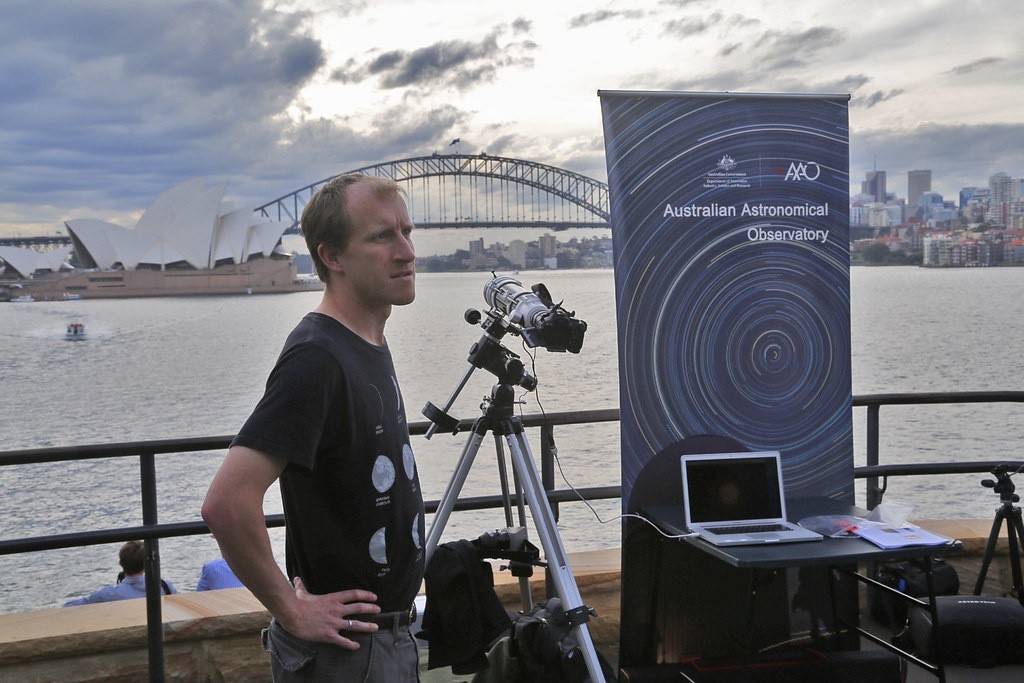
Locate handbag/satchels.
[892,596,1023,669]
[871,555,959,626]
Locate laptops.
[680,451,823,547]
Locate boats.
[66,324,85,342]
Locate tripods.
[424,309,607,683]
[974,463,1024,605]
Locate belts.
[338,601,417,633]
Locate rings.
[347,619,352,631]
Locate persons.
[195,534,246,592]
[62,540,181,607]
[200,172,429,682]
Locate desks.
[641,496,964,683]
